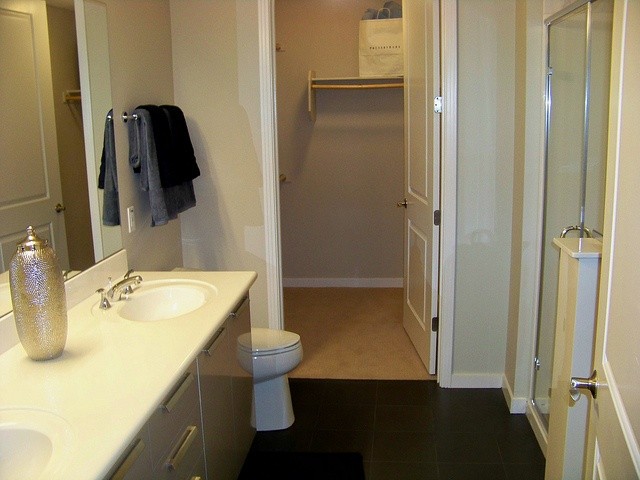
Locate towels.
[137,103,201,189]
[97,135,106,189]
[130,109,198,228]
[103,111,121,227]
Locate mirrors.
[2,1,111,345]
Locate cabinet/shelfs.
[306,69,404,121]
[0,301,207,480]
[85,262,259,480]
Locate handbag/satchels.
[358,7,404,79]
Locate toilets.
[236,332,252,376]
[252,328,304,431]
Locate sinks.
[0,428,53,480]
[118,279,218,321]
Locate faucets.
[107,275,143,300]
